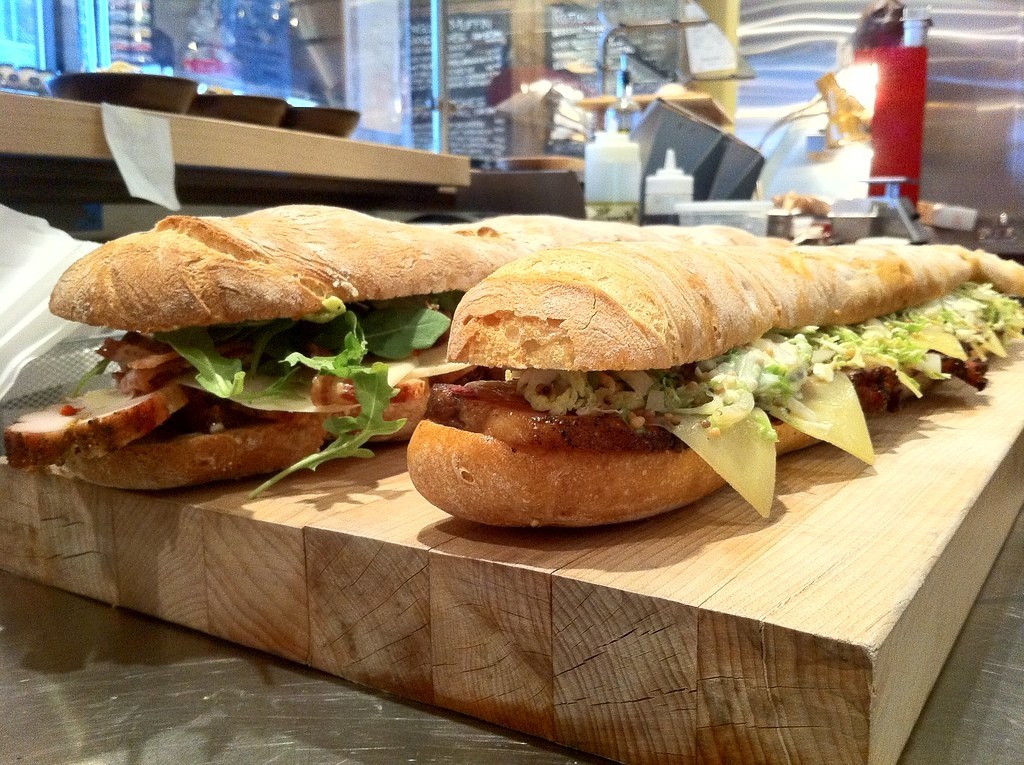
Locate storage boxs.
[676,200,770,237]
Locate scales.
[865,177,929,243]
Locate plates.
[496,155,583,185]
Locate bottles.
[915,202,978,232]
[584,110,641,223]
[816,73,871,149]
[643,149,693,225]
[603,53,641,138]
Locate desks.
[0,90,470,193]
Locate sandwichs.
[2,204,1024,528]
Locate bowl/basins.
[286,106,360,137]
[49,75,198,113]
[192,92,289,125]
[677,201,773,238]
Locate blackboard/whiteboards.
[544,0,679,158]
[410,12,511,171]
